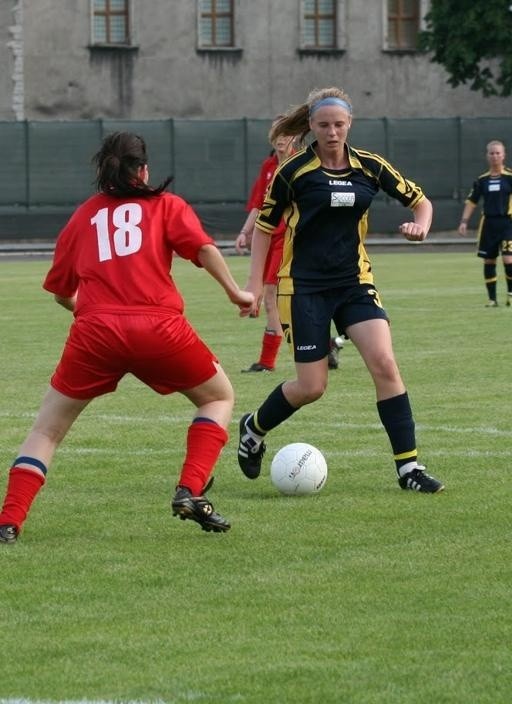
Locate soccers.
[270,442,329,495]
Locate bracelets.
[462,218,469,224]
[241,230,247,235]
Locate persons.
[0,131,254,544]
[235,114,345,373]
[238,86,445,494]
[458,140,511,306]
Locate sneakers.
[0,525,17,544]
[240,360,275,374]
[397,465,445,494]
[485,299,498,307]
[237,412,265,480]
[505,292,512,306]
[173,477,232,533]
[328,336,343,368]
